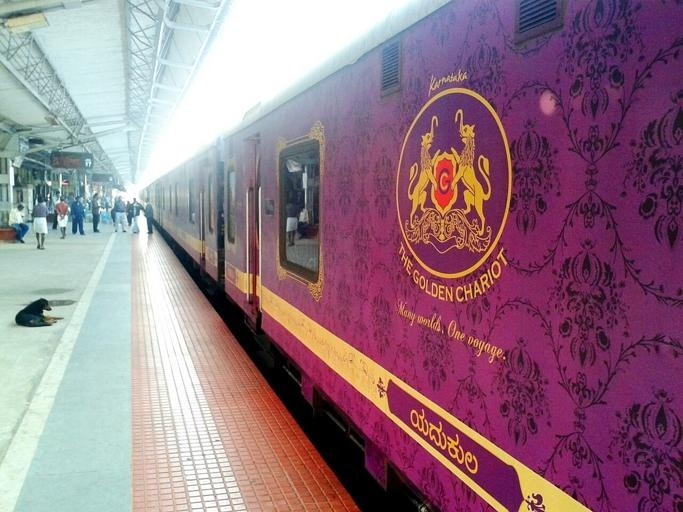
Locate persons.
[54,194,69,239]
[8,204,29,244]
[52,191,62,230]
[29,196,50,250]
[143,197,153,234]
[284,196,298,247]
[127,197,145,233]
[295,202,310,240]
[66,190,131,236]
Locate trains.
[135,1,683,512]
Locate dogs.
[15,297,64,327]
